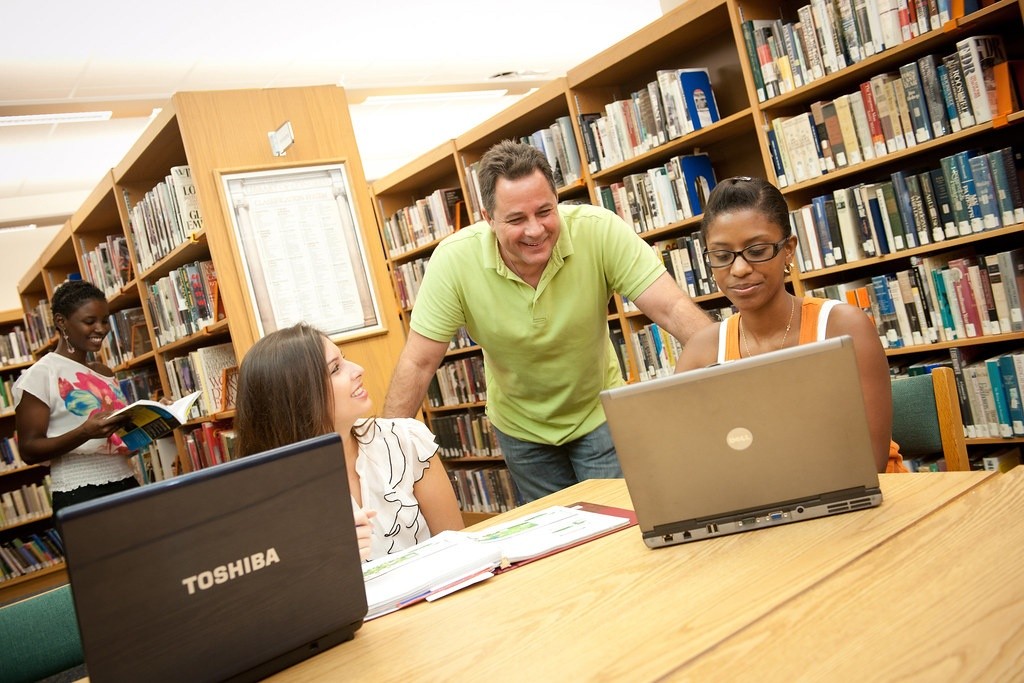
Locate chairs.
[0,583,88,683]
[890,366,970,472]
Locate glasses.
[702,236,790,269]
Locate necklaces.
[741,294,794,356]
[503,249,543,286]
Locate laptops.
[599,334,884,548]
[58,432,371,683]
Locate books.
[740,1,1024,474]
[362,498,639,623]
[105,390,204,452]
[382,66,724,511]
[0,165,241,582]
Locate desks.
[70,469,1004,683]
[666,465,1024,683]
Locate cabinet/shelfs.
[0,308,69,606]
[15,85,427,475]
[366,0,1024,445]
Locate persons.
[672,175,908,473]
[11,281,175,539]
[382,140,714,504]
[232,323,465,567]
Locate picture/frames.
[213,157,390,346]
